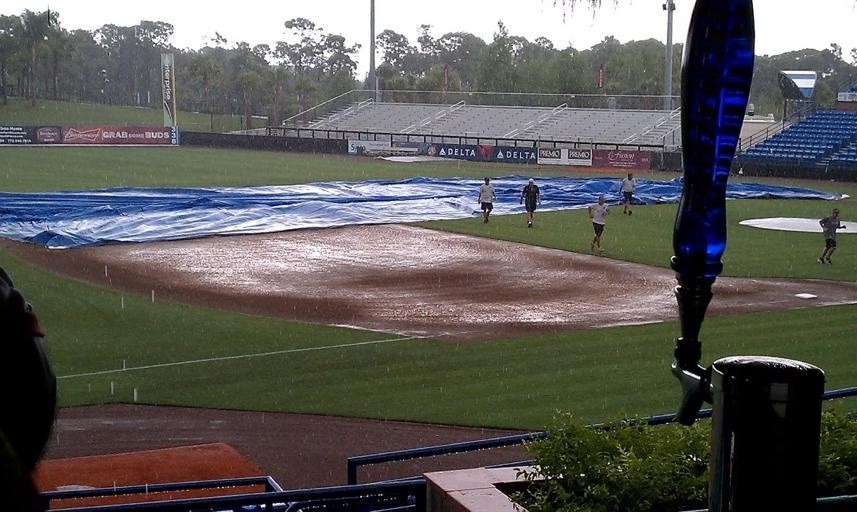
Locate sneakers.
[590,243,605,252]
[623,210,634,216]
[482,219,533,228]
[817,257,832,265]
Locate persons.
[817,208,846,265]
[588,197,610,251]
[520,179,541,228]
[619,172,636,215]
[0,265,57,511]
[745,101,755,120]
[478,177,497,224]
